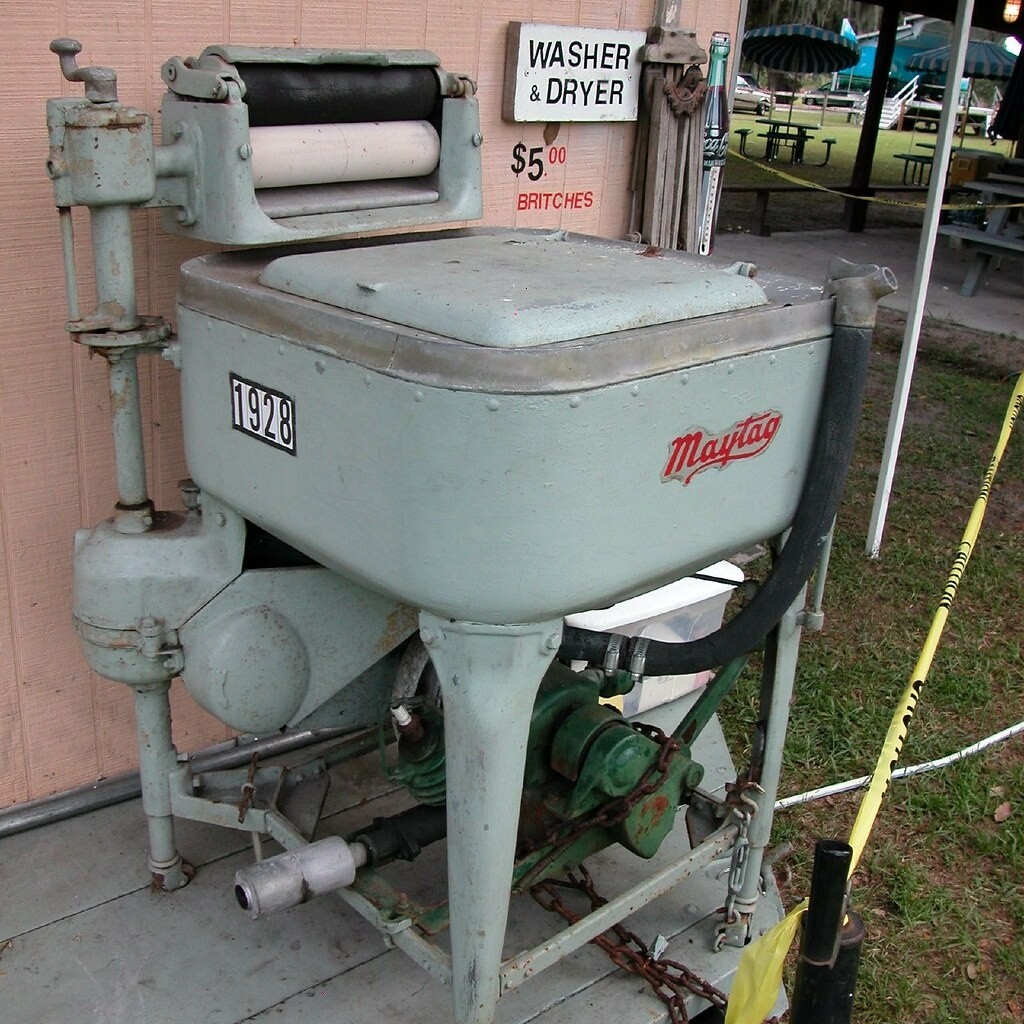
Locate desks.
[986,174,1024,184]
[961,181,1024,296]
[915,143,1004,186]
[755,118,823,162]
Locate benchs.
[895,154,1024,297]
[735,129,836,166]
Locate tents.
[835,35,969,97]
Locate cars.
[801,77,871,108]
[733,72,776,116]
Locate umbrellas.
[741,23,861,145]
[905,38,1018,149]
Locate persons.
[987,86,1004,145]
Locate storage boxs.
[950,154,986,187]
